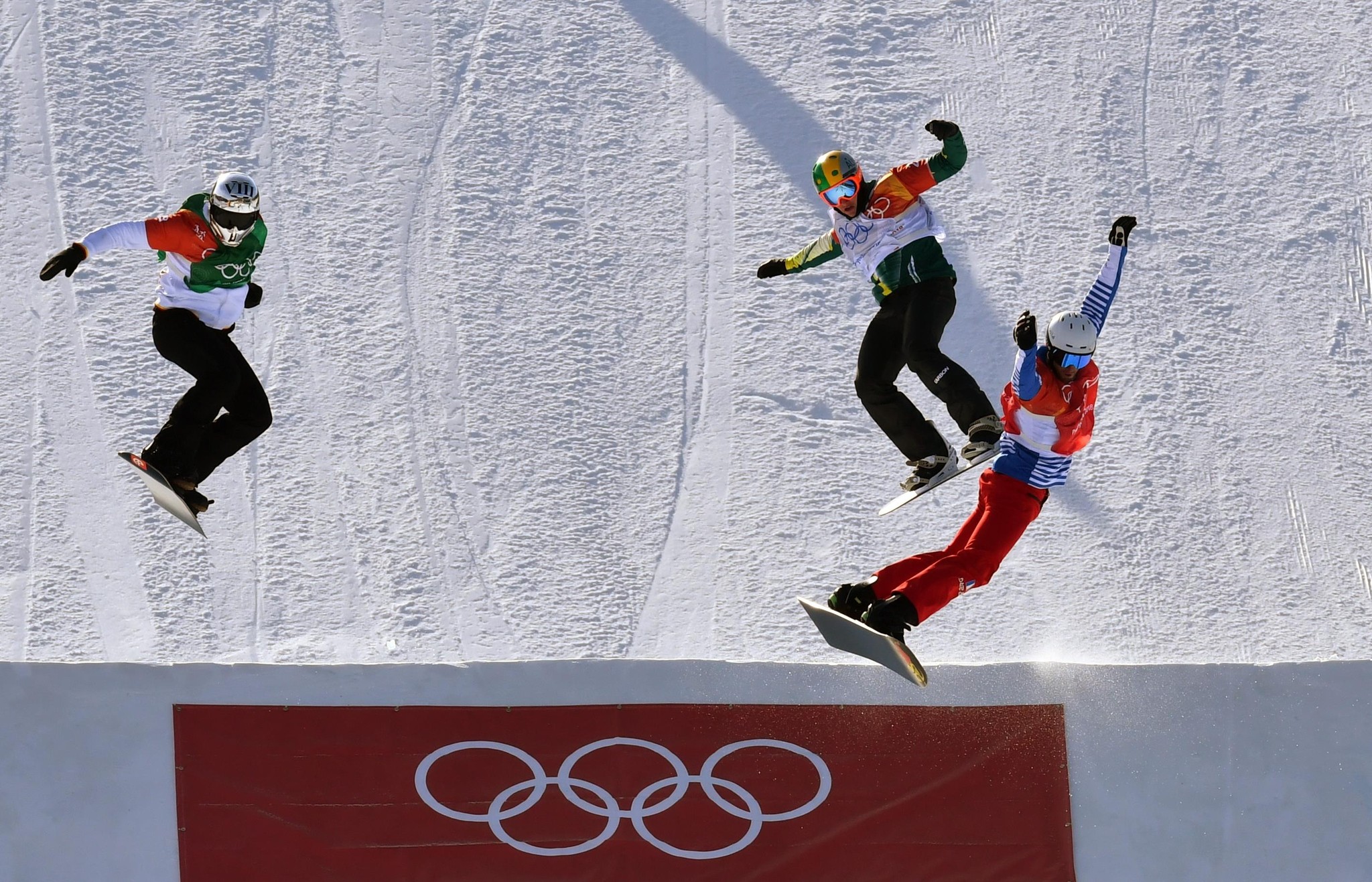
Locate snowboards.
[878,440,1004,517]
[115,450,210,541]
[793,594,930,689]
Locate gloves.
[1108,216,1137,247]
[244,282,263,310]
[925,119,957,141]
[1013,309,1037,350]
[757,259,783,279]
[40,242,87,282]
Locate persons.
[37,171,277,516]
[825,215,1144,640]
[753,117,1005,494]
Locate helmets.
[812,150,858,194]
[1048,311,1097,354]
[210,172,260,248]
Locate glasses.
[1050,346,1091,370]
[819,176,860,207]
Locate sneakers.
[904,444,959,491]
[964,420,1005,461]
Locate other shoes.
[828,582,906,646]
[140,434,209,512]
